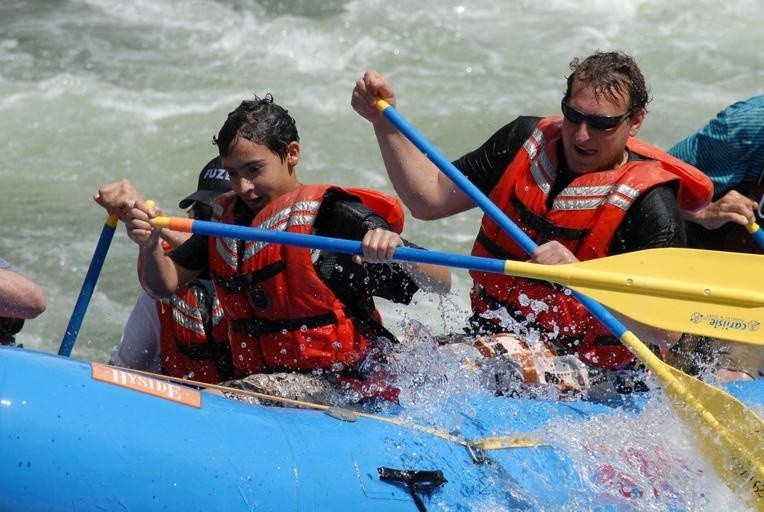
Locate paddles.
[145,201,764,343]
[371,95,764,512]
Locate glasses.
[186,203,213,220]
[561,94,633,129]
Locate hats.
[179,155,232,209]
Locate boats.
[0,344,764,512]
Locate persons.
[0,258,50,347]
[105,154,233,377]
[665,92,764,383]
[122,95,452,419]
[348,49,688,394]
